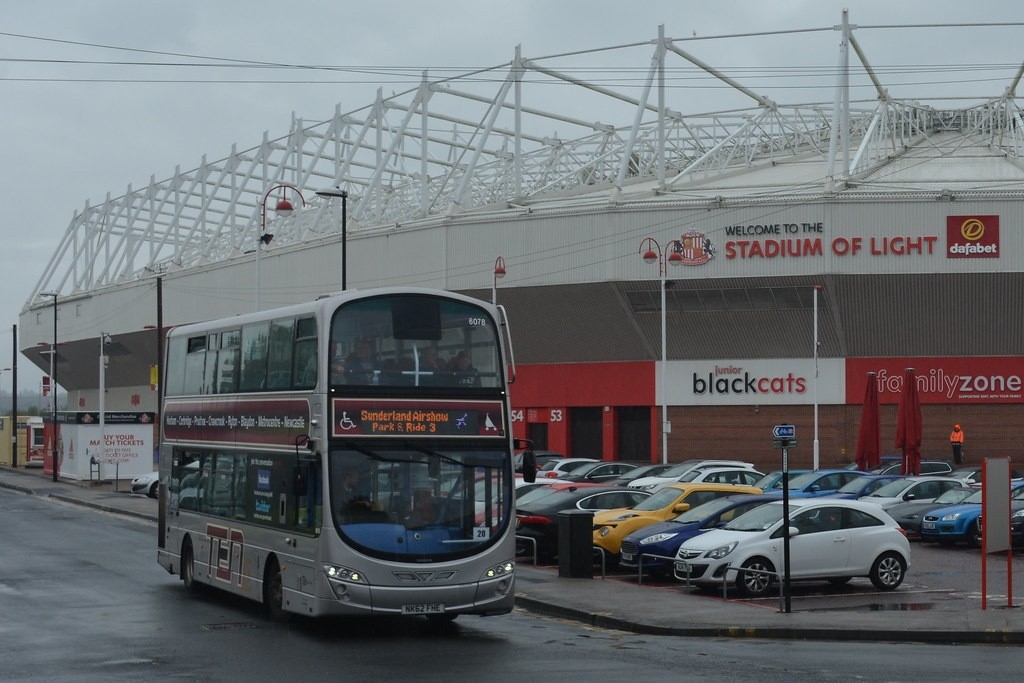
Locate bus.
[157,287,539,624]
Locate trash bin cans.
[558,509,595,579]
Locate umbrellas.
[855,372,882,473]
[894,368,922,476]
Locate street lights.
[38,291,59,481]
[315,185,348,293]
[98,332,111,485]
[638,235,685,466]
[253,182,306,311]
[492,257,506,306]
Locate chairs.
[686,493,704,510]
[613,495,635,508]
[222,356,482,393]
[653,468,665,476]
[725,472,739,484]
[794,512,814,527]
[587,495,606,509]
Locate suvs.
[868,455,956,478]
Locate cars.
[858,462,1024,554]
[674,500,911,597]
[132,461,199,500]
[369,440,900,588]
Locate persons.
[299,337,482,387]
[949,424,963,465]
